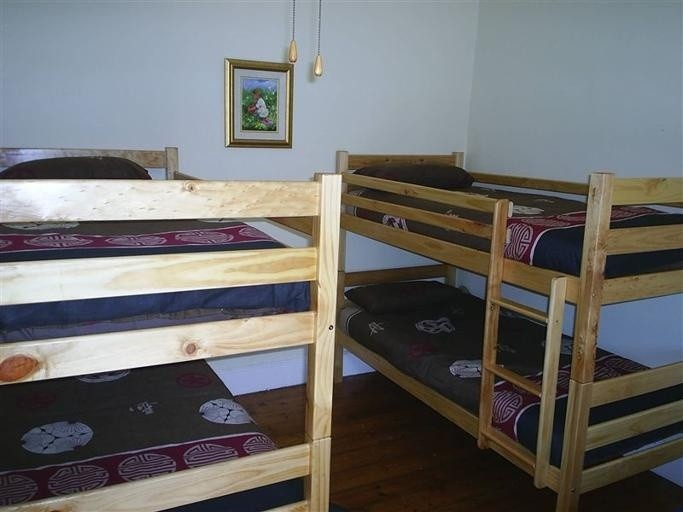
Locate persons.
[247,89,274,125]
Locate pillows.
[343,280,466,313]
[0,155,151,179]
[350,163,474,193]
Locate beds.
[333,150,682,512]
[0,147,341,511]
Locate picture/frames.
[223,59,294,150]
